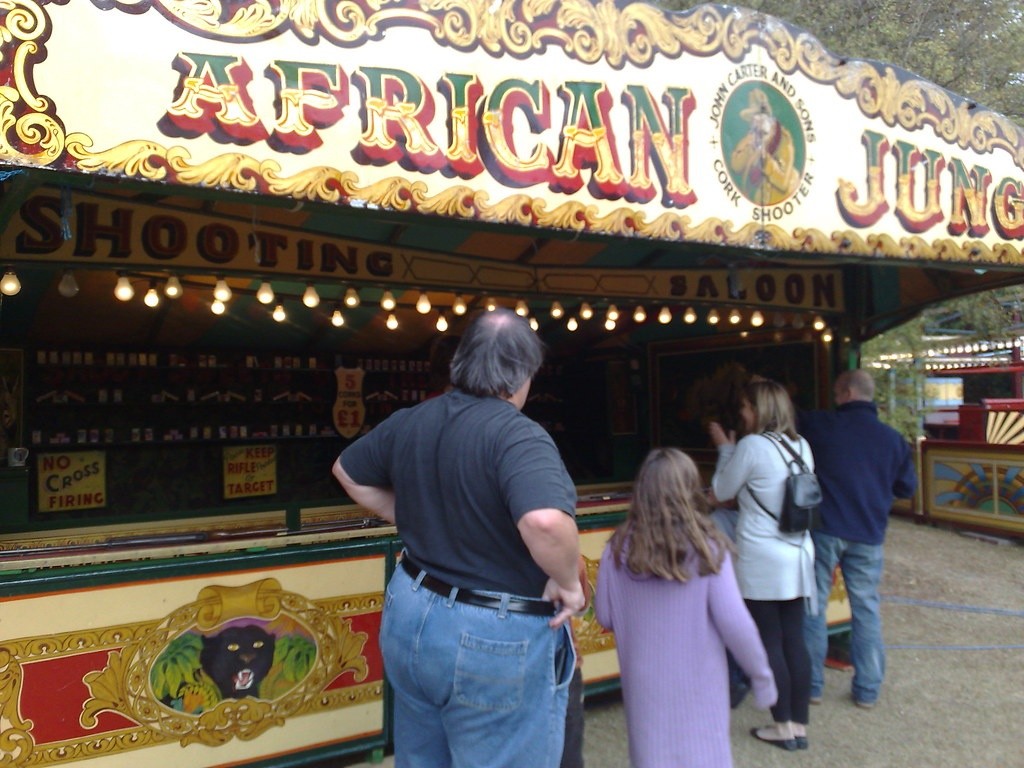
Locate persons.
[707,377,820,751]
[594,447,778,768]
[424,333,461,400]
[332,309,592,768]
[751,368,918,710]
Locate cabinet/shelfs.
[27,354,441,448]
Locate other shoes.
[797,736,807,748]
[856,700,872,708]
[809,697,822,703]
[750,728,797,750]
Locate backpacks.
[745,430,824,533]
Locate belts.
[400,551,558,616]
